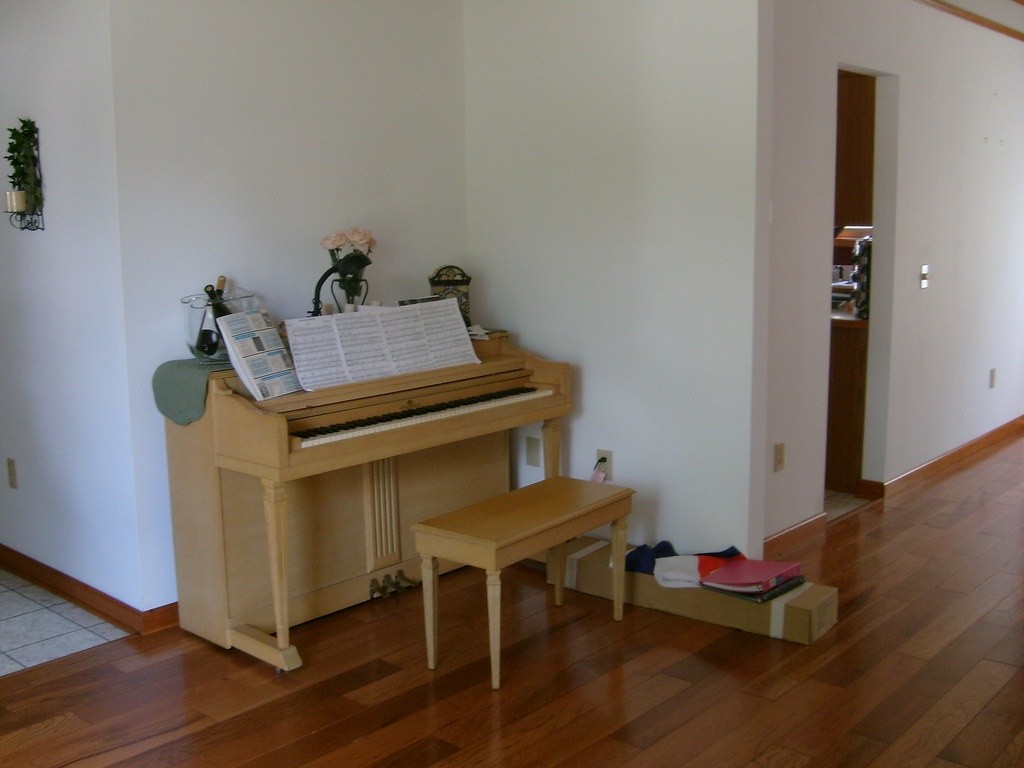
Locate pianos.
[163,324,571,670]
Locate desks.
[826,307,869,495]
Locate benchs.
[409,476,639,690]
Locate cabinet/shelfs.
[835,75,876,226]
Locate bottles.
[196,276,226,355]
[205,284,234,344]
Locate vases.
[344,304,357,313]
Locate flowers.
[320,225,377,305]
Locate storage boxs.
[467,328,510,358]
[545,534,839,646]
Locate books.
[215,308,303,401]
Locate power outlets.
[526,436,541,468]
[596,449,613,481]
[773,440,785,472]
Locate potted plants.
[2,117,44,214]
